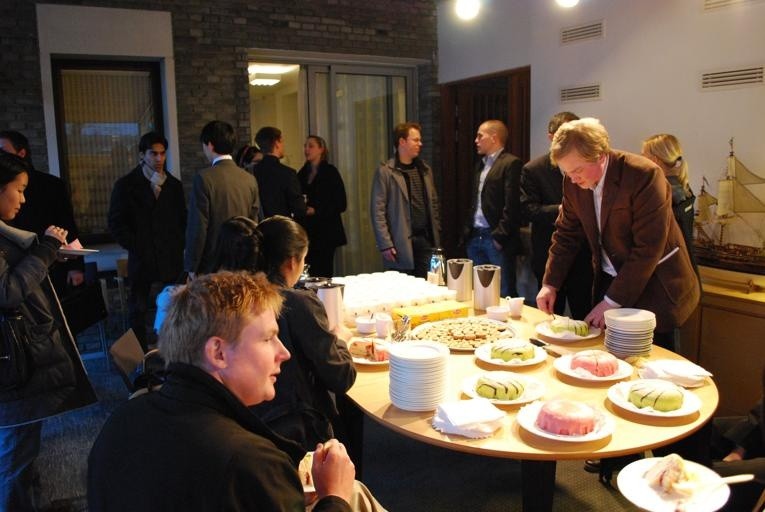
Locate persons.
[0,149,98,512]
[250,215,357,451]
[89,270,387,512]
[370,122,443,280]
[209,214,263,272]
[522,111,590,326]
[236,143,262,171]
[185,120,260,281]
[1,130,85,288]
[640,133,700,277]
[107,130,189,336]
[723,395,765,462]
[247,127,303,218]
[535,117,702,473]
[298,134,348,277]
[458,118,521,298]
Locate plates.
[666,264,761,420]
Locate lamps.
[67,277,115,371]
[117,258,145,338]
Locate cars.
[506,296,525,320]
[375,312,394,339]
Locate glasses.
[250,71,281,86]
[455,0,480,20]
[557,0,577,10]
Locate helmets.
[603,308,656,359]
[514,401,616,442]
[607,379,702,419]
[536,321,601,341]
[617,455,730,512]
[461,371,546,406]
[554,351,634,382]
[389,338,451,412]
[346,336,390,366]
[412,316,520,352]
[475,340,548,368]
[58,246,100,256]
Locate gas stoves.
[491,342,534,362]
[537,399,594,436]
[477,376,525,401]
[350,340,386,362]
[571,349,619,377]
[643,452,684,495]
[551,316,588,336]
[628,379,684,411]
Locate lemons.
[446,258,474,302]
[430,247,446,286]
[317,279,345,331]
[304,277,332,292]
[474,265,501,311]
[298,263,311,283]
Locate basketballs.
[416,319,512,349]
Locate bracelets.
[486,306,509,322]
[356,315,376,333]
